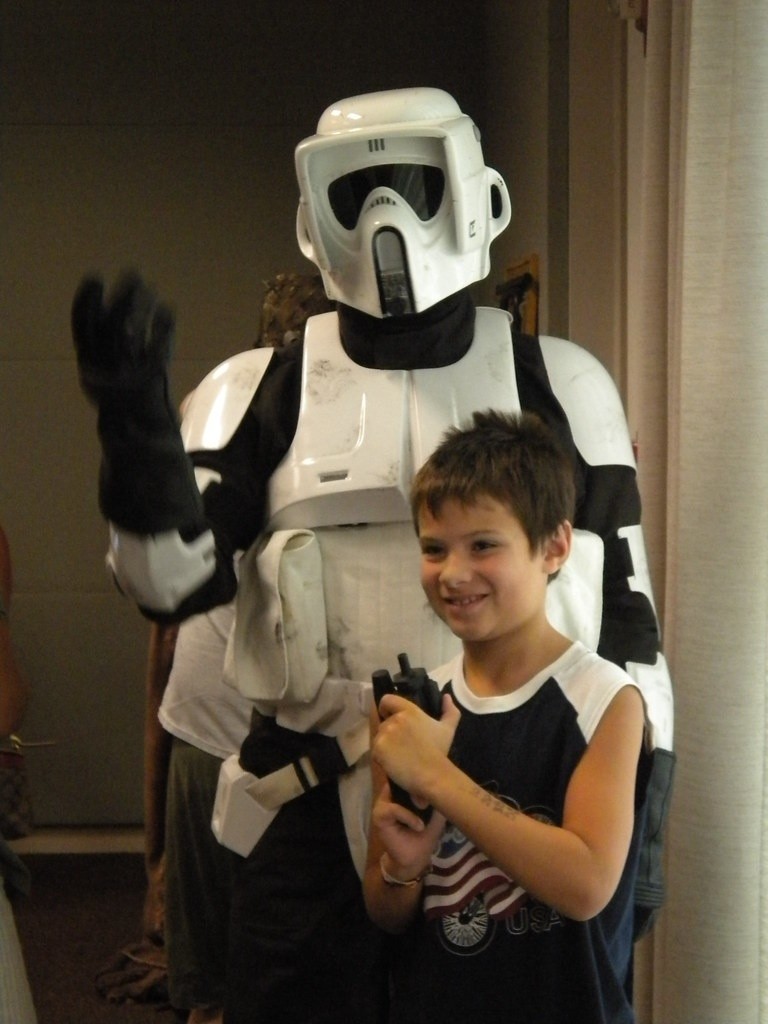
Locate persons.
[363,408,647,1024]
[72,87,676,1024]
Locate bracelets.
[380,852,433,889]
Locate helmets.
[296,87,511,319]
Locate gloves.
[633,751,677,943]
[70,268,206,537]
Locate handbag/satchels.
[0,733,34,841]
